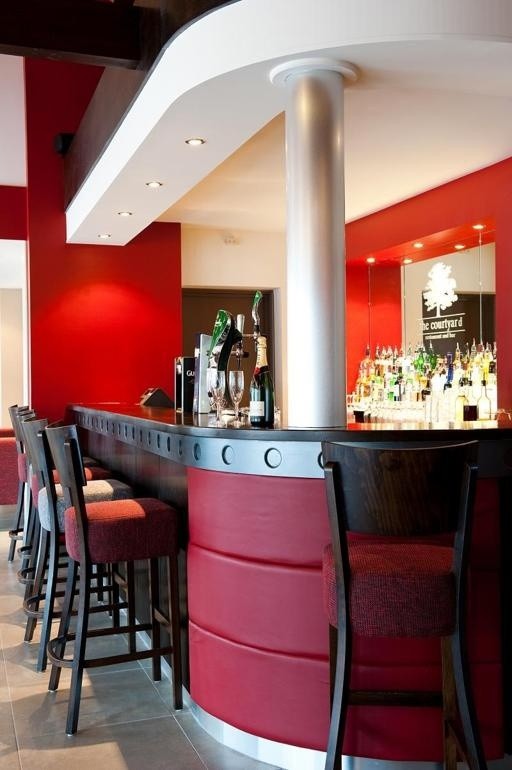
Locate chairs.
[314,433,490,770]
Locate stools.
[8,405,190,738]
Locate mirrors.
[366,240,497,371]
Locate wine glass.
[206,368,246,427]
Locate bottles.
[249,336,276,429]
[347,339,497,424]
